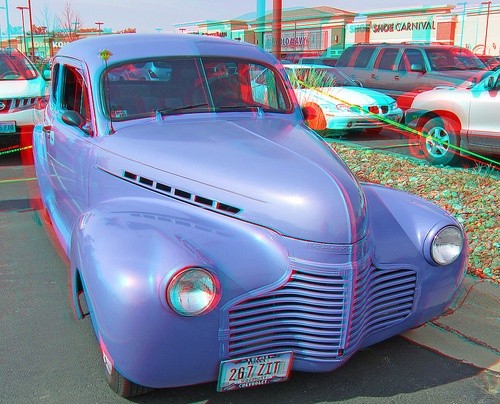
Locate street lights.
[95,22,103,36]
[16,6,28,54]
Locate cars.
[0,47,52,137]
[32,33,469,404]
[250,64,403,137]
[107,59,238,80]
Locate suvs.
[279,51,337,67]
[405,61,500,168]
[334,40,495,117]
